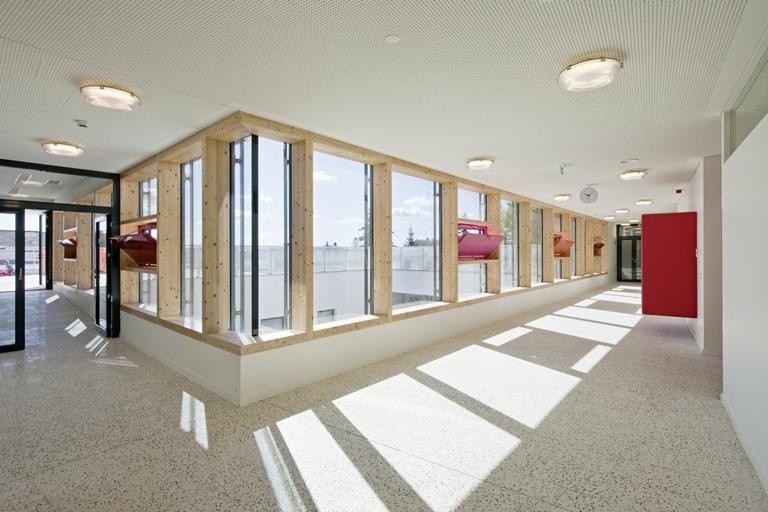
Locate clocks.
[580,187,598,203]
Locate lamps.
[466,156,495,170]
[41,140,86,158]
[80,80,140,111]
[618,169,647,180]
[605,200,652,226]
[556,52,623,92]
[553,193,571,201]
